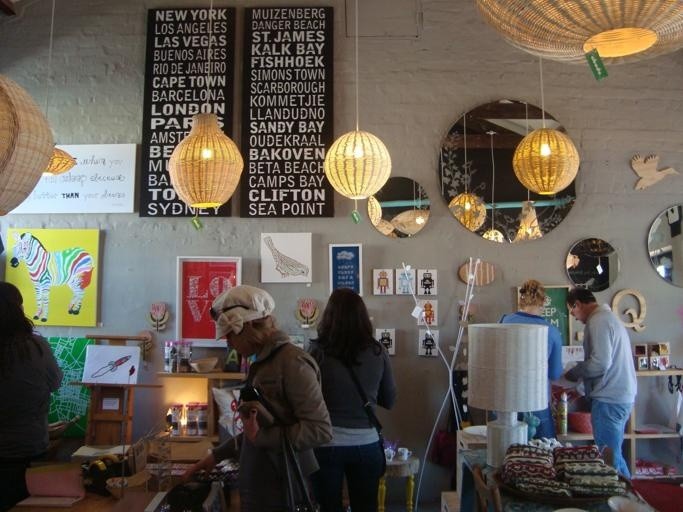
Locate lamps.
[465,321,551,469]
[511,55,581,197]
[168,0,245,211]
[0,72,56,218]
[447,101,544,242]
[160,374,210,437]
[43,0,78,178]
[367,195,431,238]
[472,0,683,66]
[323,1,392,200]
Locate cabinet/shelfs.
[556,369,683,481]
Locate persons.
[489,279,563,440]
[181,284,334,512]
[1,279,65,512]
[563,286,637,484]
[306,287,397,511]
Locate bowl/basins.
[189,357,218,373]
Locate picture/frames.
[328,241,364,298]
[174,255,243,349]
[633,342,670,371]
[515,285,573,347]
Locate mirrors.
[367,177,431,239]
[438,98,582,245]
[565,236,621,292]
[647,204,683,288]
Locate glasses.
[568,302,577,317]
[209,303,249,321]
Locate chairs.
[469,464,503,512]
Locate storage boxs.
[440,429,487,512]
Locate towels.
[501,443,627,500]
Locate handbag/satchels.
[371,426,387,477]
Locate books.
[15,457,86,508]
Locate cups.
[397,447,412,461]
[384,448,395,462]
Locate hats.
[207,283,275,341]
